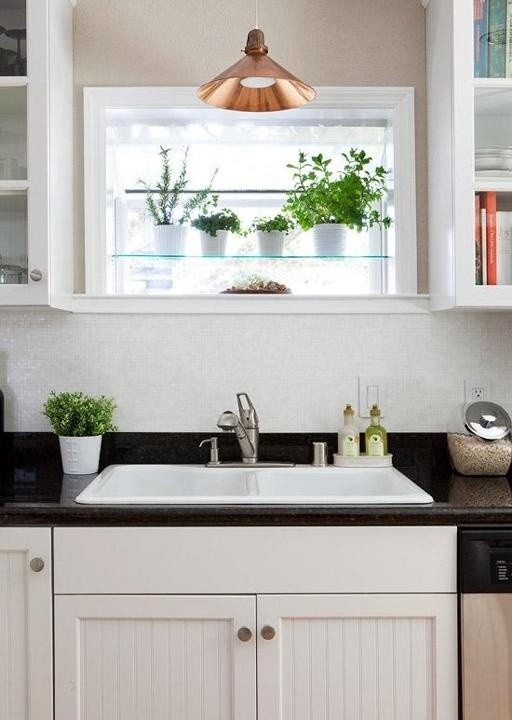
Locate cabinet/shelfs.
[1,522,54,718]
[49,518,460,720]
[424,0,512,313]
[1,1,80,316]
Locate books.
[474,190,497,284]
[475,1,512,78]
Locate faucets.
[216,391,260,465]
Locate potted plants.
[38,386,123,478]
[127,123,400,263]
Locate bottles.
[446,402,511,476]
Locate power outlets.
[464,376,497,409]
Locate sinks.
[72,461,250,505]
[251,464,434,508]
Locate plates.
[473,145,512,182]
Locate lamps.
[196,0,318,116]
